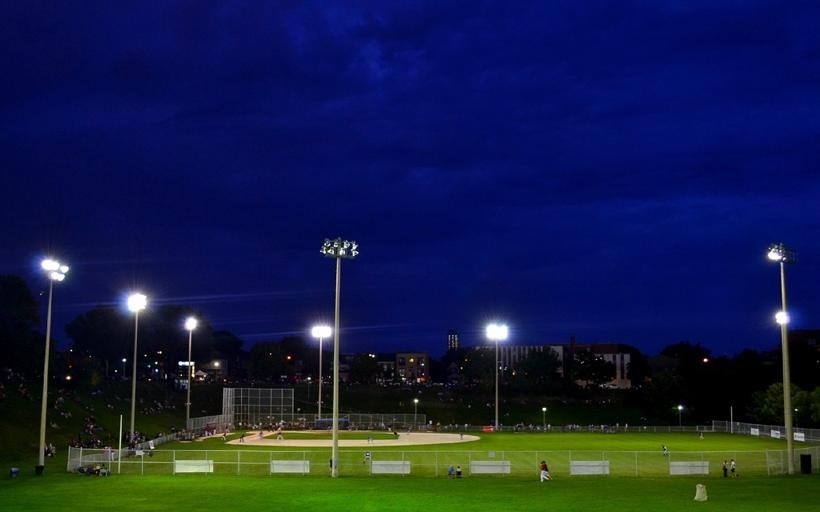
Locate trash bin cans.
[800,454,811,474]
[35,465,44,475]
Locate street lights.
[768,243,795,474]
[311,325,332,417]
[414,399,419,425]
[35,259,70,476]
[186,317,197,429]
[677,405,684,427]
[127,290,147,434]
[485,323,509,426]
[541,407,547,425]
[320,237,360,479]
